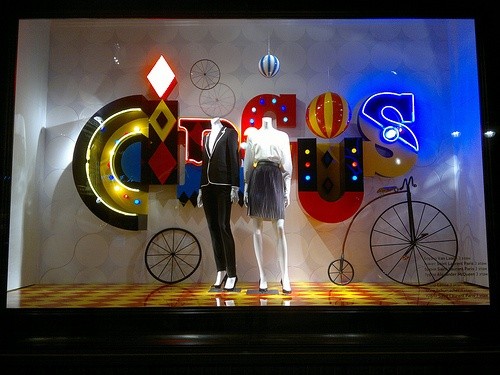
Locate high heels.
[258,279,268,293]
[223,275,238,291]
[211,273,228,290]
[280,277,292,293]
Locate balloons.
[305,91,352,139]
[258,55,280,78]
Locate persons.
[243,116,293,294]
[196,117,239,292]
